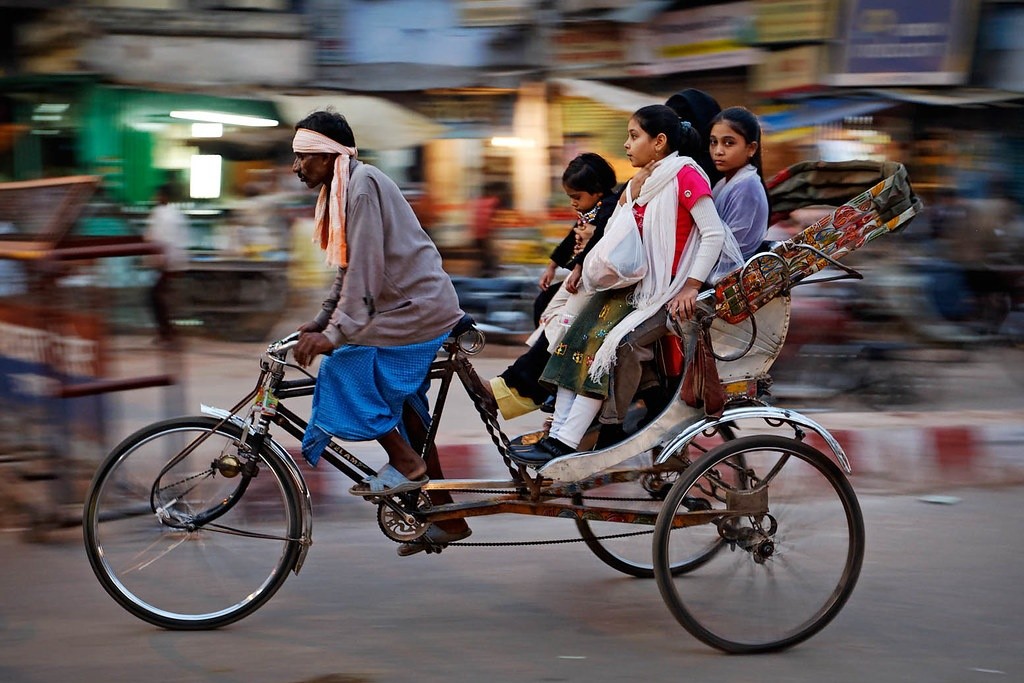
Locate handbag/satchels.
[582,180,649,293]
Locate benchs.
[532,234,790,381]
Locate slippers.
[397,521,473,556]
[348,462,430,495]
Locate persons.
[137,182,189,351]
[293,113,472,558]
[472,87,769,465]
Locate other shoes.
[457,355,498,418]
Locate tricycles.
[79,159,929,658]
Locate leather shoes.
[504,439,567,466]
